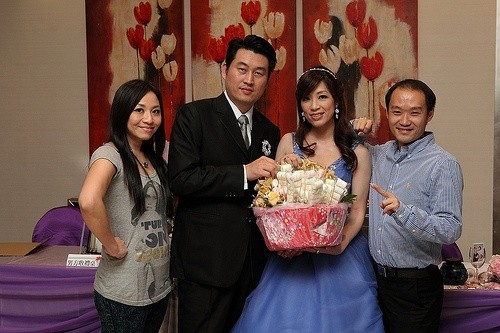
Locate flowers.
[261,139,272,156]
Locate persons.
[168,34,281,333]
[274,64,373,333]
[77,79,174,333]
[352,80,464,333]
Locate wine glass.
[469,243,487,290]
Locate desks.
[0,239,500,333]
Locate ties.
[238,115,249,151]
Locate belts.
[375,264,438,278]
